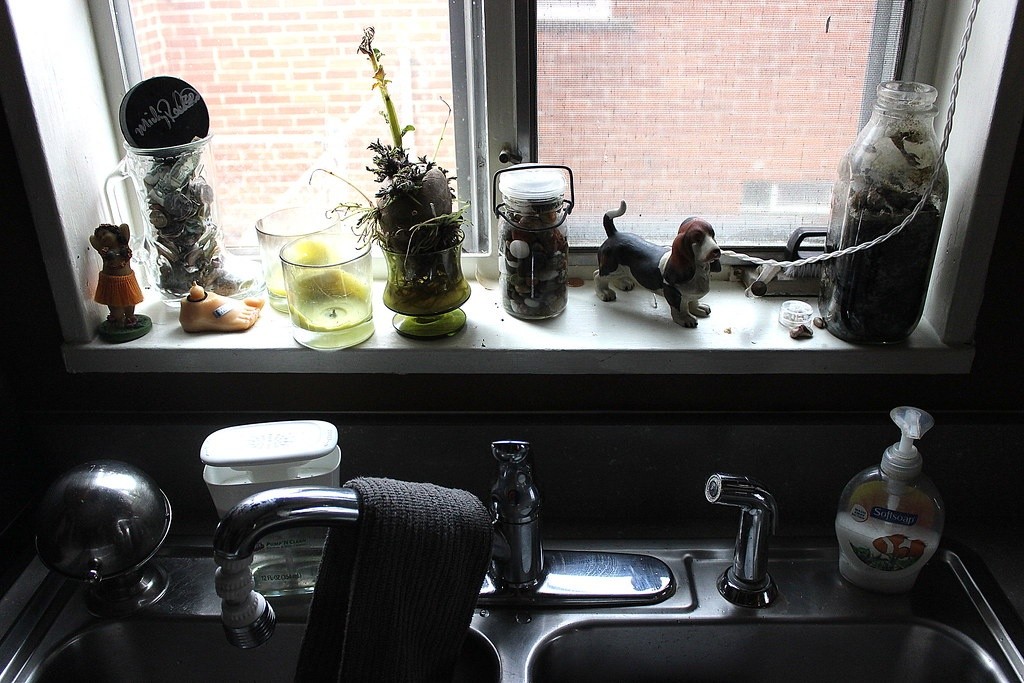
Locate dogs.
[593,199,722,329]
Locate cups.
[255,206,341,314]
[99,134,232,308]
[279,231,374,352]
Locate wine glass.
[379,228,472,340]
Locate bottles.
[818,79,950,346]
[491,163,571,321]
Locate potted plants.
[306,24,476,337]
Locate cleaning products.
[834,404,947,595]
[200,419,343,599]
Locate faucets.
[212,437,544,651]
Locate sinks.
[0,589,508,683]
[517,596,1024,683]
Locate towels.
[295,476,495,683]
